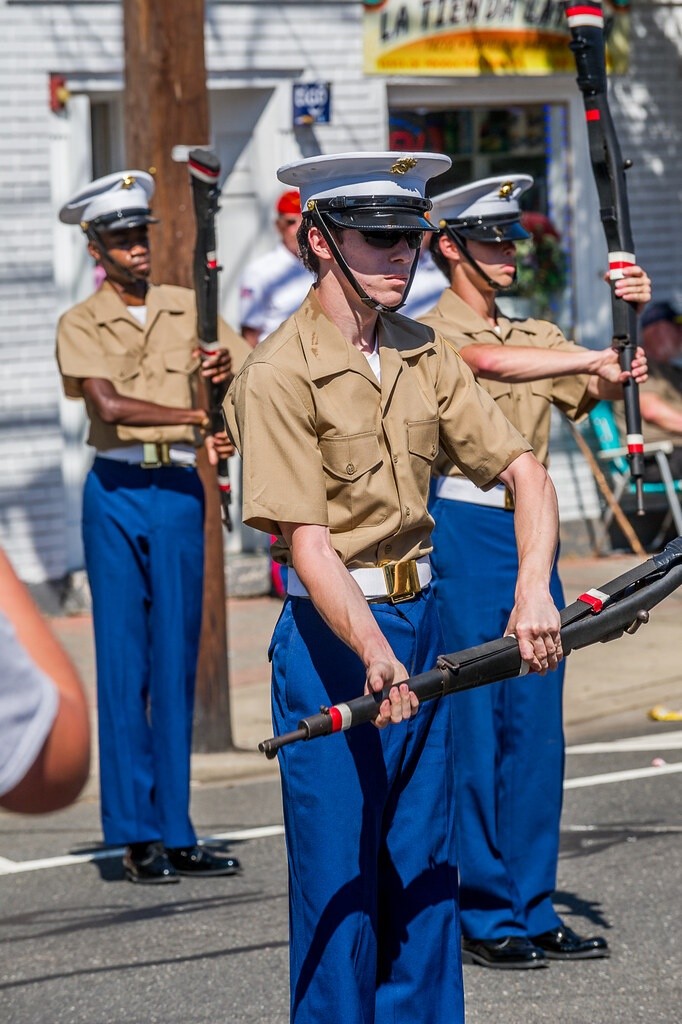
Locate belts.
[287,554,434,598]
[97,444,195,464]
[436,475,507,508]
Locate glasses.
[357,229,424,249]
[280,218,296,225]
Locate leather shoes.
[124,845,179,884]
[534,926,608,958]
[462,938,547,969]
[169,847,239,876]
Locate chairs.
[590,400,682,553]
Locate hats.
[276,152,453,230]
[59,171,158,230]
[278,192,300,214]
[639,299,682,327]
[426,174,534,241]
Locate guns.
[561,0,655,519]
[187,146,234,534]
[257,535,682,762]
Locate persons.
[49,165,252,887]
[221,189,317,351]
[0,547,91,816]
[408,170,652,976]
[609,297,682,485]
[218,146,567,1024]
